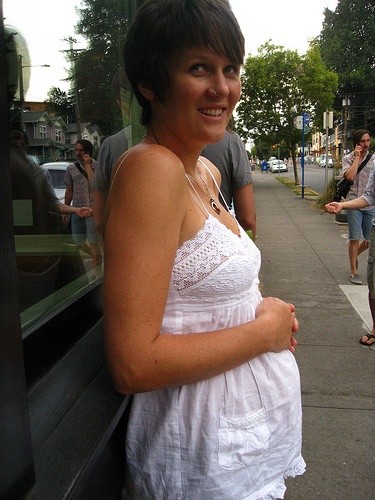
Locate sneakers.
[356,257,358,269]
[349,274,362,284]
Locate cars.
[259,156,288,173]
[299,153,334,168]
[41,160,77,226]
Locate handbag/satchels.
[335,177,352,201]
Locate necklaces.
[189,169,222,215]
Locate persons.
[341,129,375,284]
[100,0,305,500]
[93,126,257,230]
[324,160,375,346]
[63,140,100,281]
[23,130,94,220]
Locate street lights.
[17,54,50,132]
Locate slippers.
[360,333,375,345]
